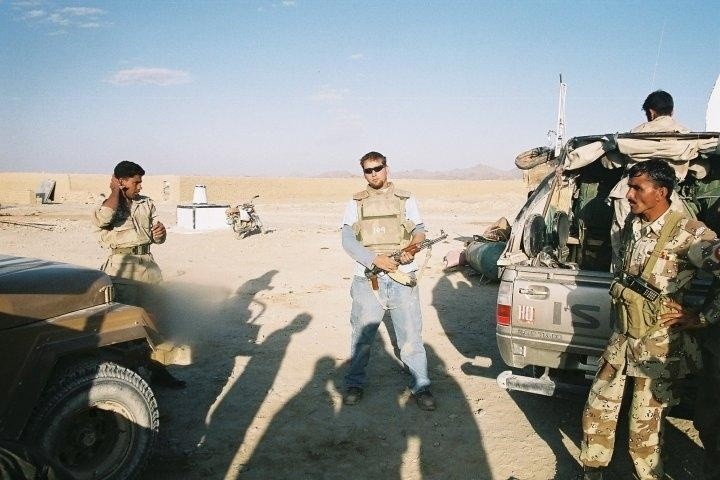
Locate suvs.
[0,252,164,479]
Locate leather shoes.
[148,372,186,388]
[344,387,363,405]
[412,388,436,411]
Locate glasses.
[362,164,386,174]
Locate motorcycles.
[226,194,263,240]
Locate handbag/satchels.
[608,277,660,339]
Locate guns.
[365,233,447,291]
[565,131,716,149]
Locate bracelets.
[699,311,706,325]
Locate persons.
[342,151,436,411]
[573,158,720,480]
[604,90,712,274]
[91,161,186,387]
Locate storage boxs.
[177,204,231,231]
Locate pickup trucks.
[491,126,720,406]
